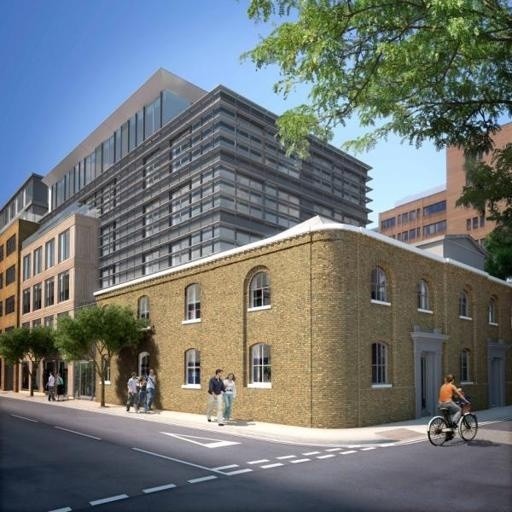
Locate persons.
[145,368,157,411]
[136,376,150,414]
[205,368,226,426]
[436,373,471,442]
[126,372,139,411]
[56,373,66,401]
[45,372,57,402]
[222,372,236,421]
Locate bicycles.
[427,396,477,446]
